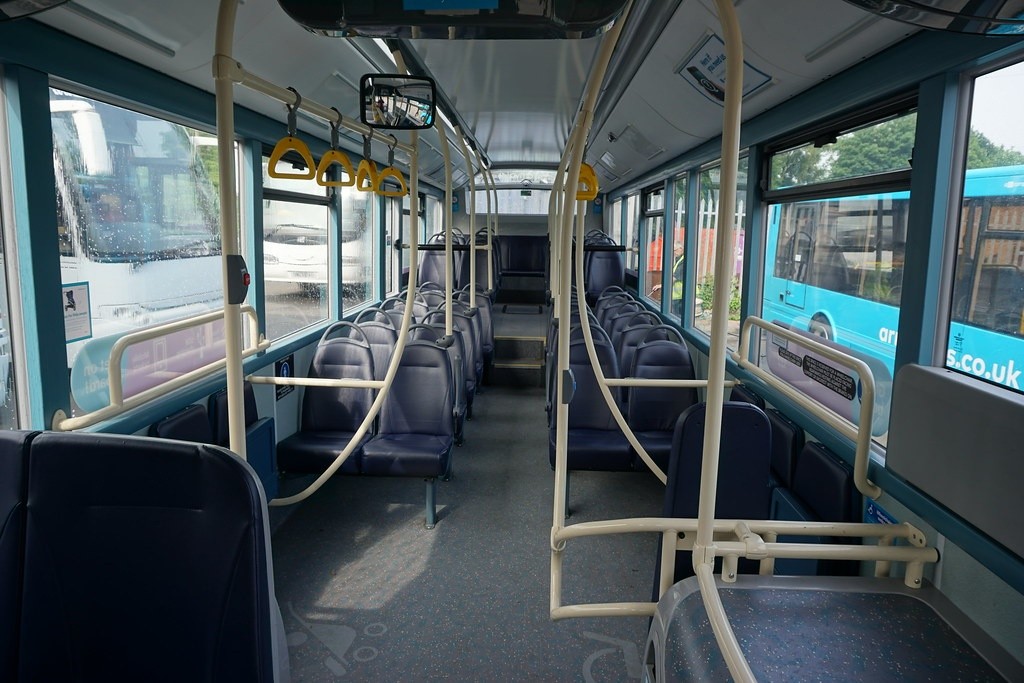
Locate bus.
[259,160,441,300]
[1,82,246,434]
[759,164,1023,449]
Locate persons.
[652,241,685,319]
[377,97,385,113]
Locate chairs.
[547,230,774,606]
[779,232,1023,334]
[0,225,506,683]
